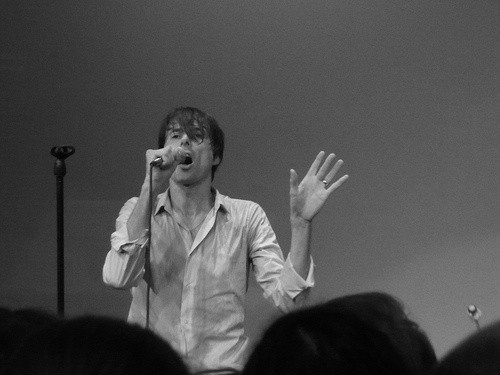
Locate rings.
[323,180,328,186]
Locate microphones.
[467,304,482,321]
[150,146,186,166]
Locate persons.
[1,306,191,375]
[241,292,500,375]
[102,107,349,375]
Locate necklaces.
[190,216,206,231]
